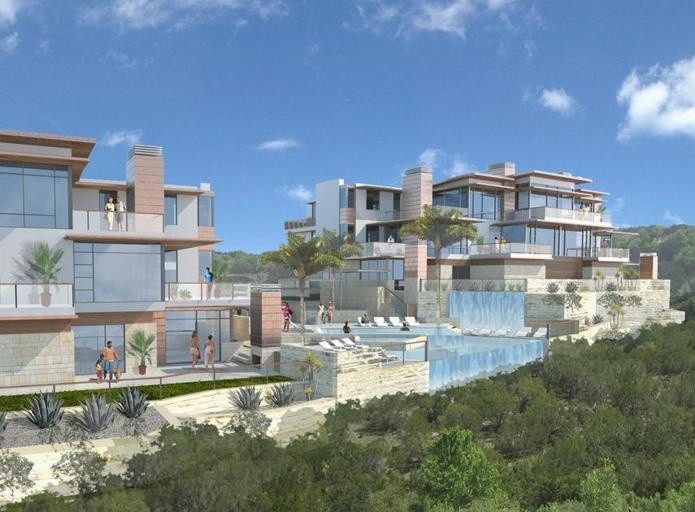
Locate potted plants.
[210,258,230,299]
[12,241,64,307]
[178,288,193,299]
[124,329,157,374]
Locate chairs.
[318,335,368,352]
[357,316,420,328]
[289,320,313,332]
[470,326,551,338]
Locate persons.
[189,330,201,368]
[325,301,334,323]
[600,238,609,249]
[400,321,409,332]
[494,235,506,255]
[203,267,214,300]
[204,335,216,373]
[282,302,291,332]
[95,341,121,384]
[577,204,590,222]
[387,234,395,243]
[317,301,325,326]
[105,196,127,232]
[361,312,370,328]
[343,321,351,333]
